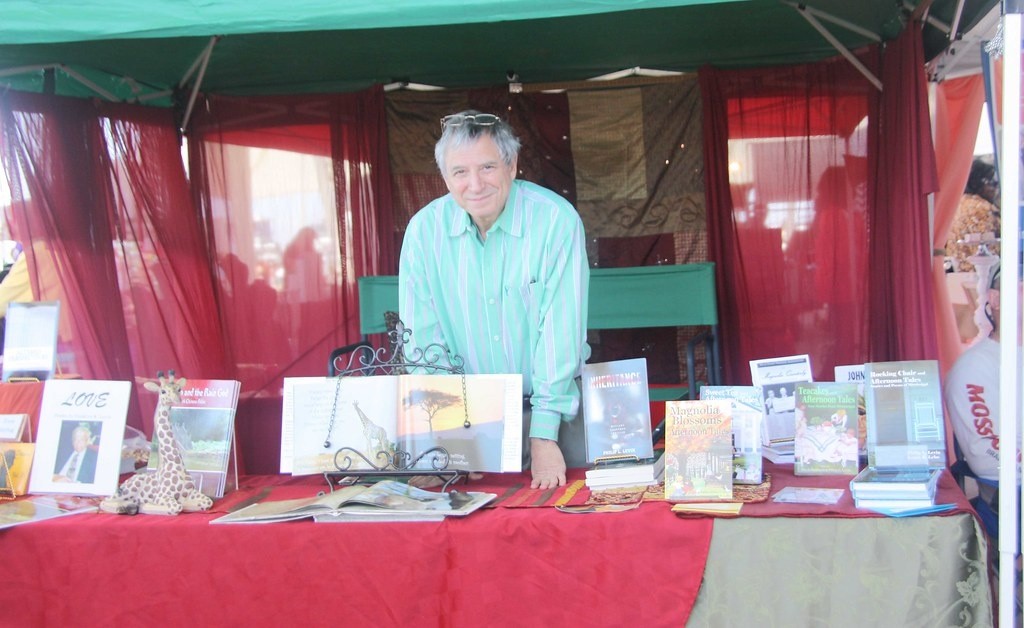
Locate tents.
[1,1,1023,628]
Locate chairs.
[951,431,1023,616]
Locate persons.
[397,112,590,490]
[283,226,332,364]
[0,199,83,374]
[52,426,97,485]
[765,387,795,415]
[945,261,1022,517]
[944,156,1003,271]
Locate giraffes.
[351,400,387,452]
[100,368,214,516]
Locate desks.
[0,473,1001,628]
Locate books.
[795,381,859,476]
[665,401,735,500]
[834,360,948,508]
[209,479,497,525]
[700,386,762,484]
[146,378,241,500]
[579,358,665,490]
[280,374,524,473]
[29,379,134,497]
[748,355,814,455]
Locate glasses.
[440,115,501,132]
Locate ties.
[66,453,78,483]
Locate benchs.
[327,262,721,405]
